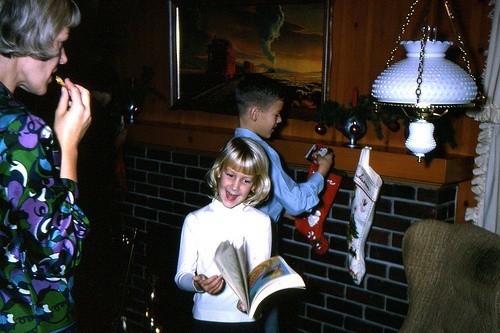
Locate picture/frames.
[167,0,332,114]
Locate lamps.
[371,0,477,163]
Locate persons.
[0,0,93,333]
[234,72,334,333]
[175,137,273,333]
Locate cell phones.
[304,144,330,160]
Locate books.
[213,237,306,319]
